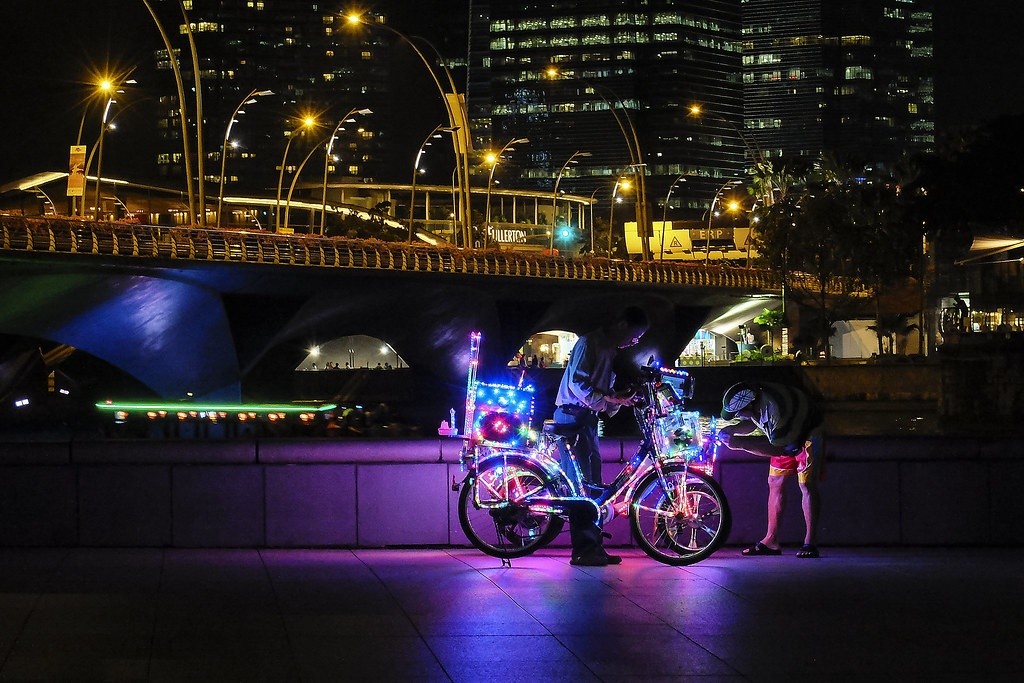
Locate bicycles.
[457,369,734,568]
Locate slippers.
[796,543,820,558]
[741,542,782,555]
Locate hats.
[719,380,761,422]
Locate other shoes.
[569,545,622,566]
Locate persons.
[325,362,340,370]
[520,354,529,368]
[554,306,648,567]
[539,357,545,368]
[566,358,568,366]
[716,380,826,558]
[953,295,968,332]
[532,355,538,367]
[796,348,810,365]
[375,363,392,370]
[345,362,349,369]
[871,353,877,359]
[312,363,317,369]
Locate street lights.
[706,180,743,268]
[276,116,317,233]
[345,16,473,248]
[688,104,771,210]
[94,79,112,220]
[321,107,374,235]
[546,67,652,263]
[217,87,275,228]
[409,122,461,243]
[608,162,647,258]
[549,150,593,256]
[484,137,529,251]
